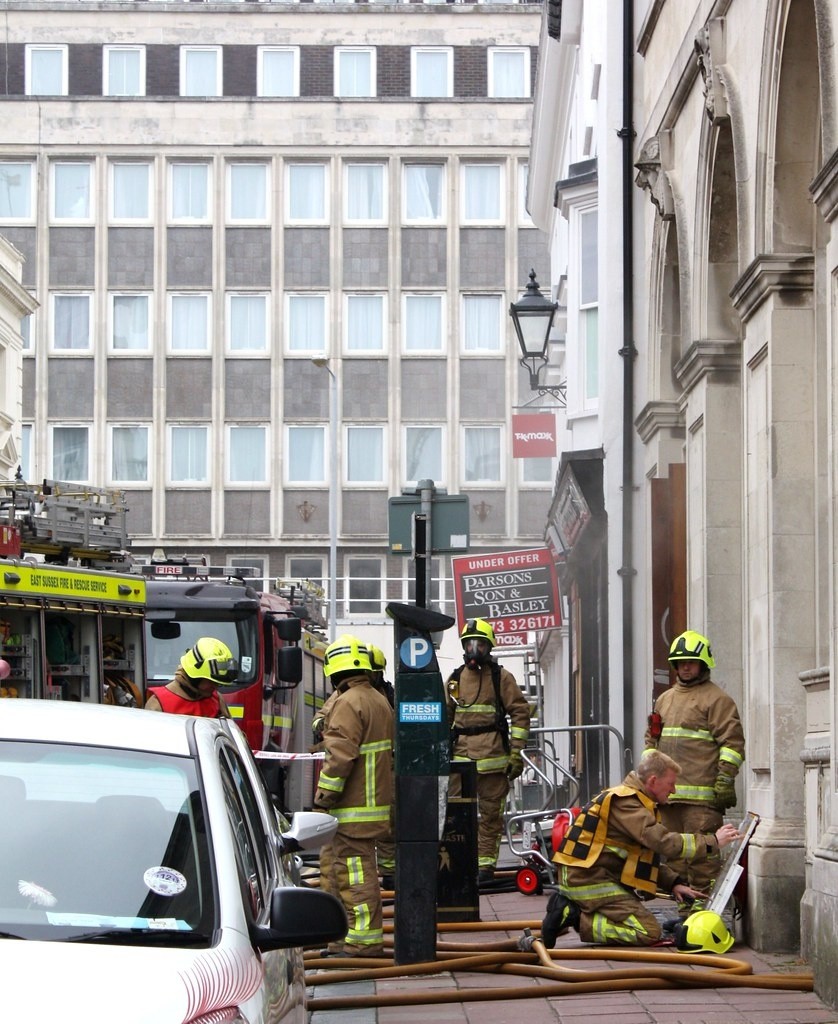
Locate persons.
[309,634,396,965]
[260,729,289,814]
[143,636,250,745]
[537,749,745,951]
[641,631,747,935]
[442,617,530,883]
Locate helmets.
[461,619,496,648]
[668,630,716,672]
[180,637,234,684]
[324,634,387,677]
[676,909,735,954]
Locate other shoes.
[326,951,359,958]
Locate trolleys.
[504,747,581,895]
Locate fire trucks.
[134,555,333,817]
[0,482,149,707]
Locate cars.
[0,697,349,1024]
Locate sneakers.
[542,892,579,949]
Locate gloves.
[311,717,327,739]
[715,772,738,814]
[507,744,523,783]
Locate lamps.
[510,268,558,390]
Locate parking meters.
[384,601,457,966]
